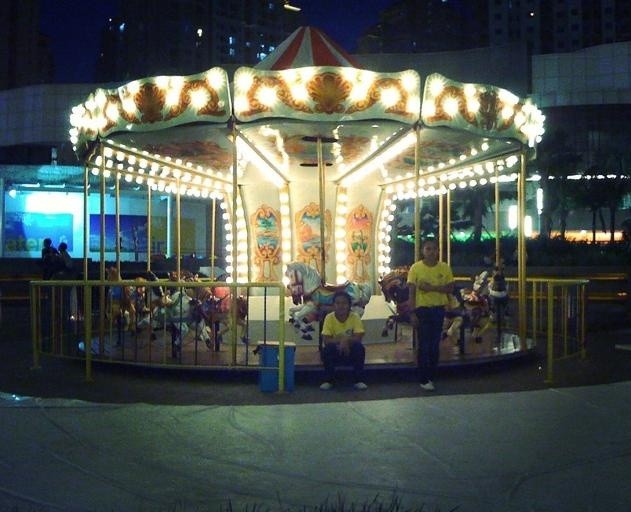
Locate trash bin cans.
[257,340,297,393]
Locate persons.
[41,238,71,264]
[320,291,368,391]
[406,237,455,391]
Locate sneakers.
[420,380,435,391]
[320,382,333,390]
[354,382,367,391]
